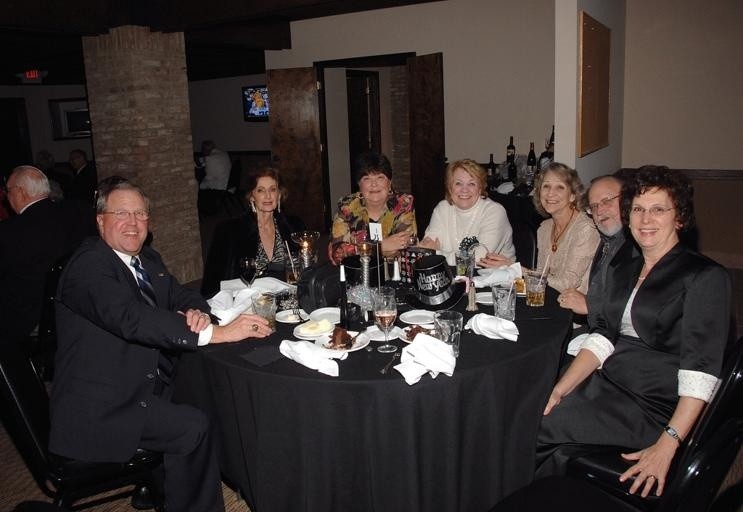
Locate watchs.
[664,426,682,446]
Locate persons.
[199,166,309,298]
[327,149,417,267]
[58,149,97,202]
[197,140,232,216]
[0,165,90,339]
[477,160,600,296]
[538,164,733,499]
[417,157,517,269]
[46,174,273,512]
[557,175,642,366]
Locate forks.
[292,306,304,323]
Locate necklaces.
[551,207,575,253]
[637,275,646,280]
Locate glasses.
[628,204,679,219]
[584,193,620,216]
[3,183,25,193]
[98,209,152,223]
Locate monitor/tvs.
[242,84,269,123]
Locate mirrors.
[46,98,93,140]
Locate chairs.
[566,334,741,511]
[205,214,310,276]
[0,338,164,511]
[510,221,535,271]
[37,267,59,346]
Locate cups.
[403,236,419,248]
[251,292,276,333]
[522,266,548,307]
[454,252,474,277]
[433,309,462,358]
[238,258,256,286]
[285,254,300,283]
[491,281,516,321]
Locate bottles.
[508,154,517,180]
[389,255,401,281]
[381,257,390,280]
[466,281,477,311]
[527,141,535,171]
[486,152,494,180]
[549,124,553,144]
[506,135,514,164]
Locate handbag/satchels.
[404,254,467,313]
[296,263,344,314]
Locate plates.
[399,309,436,325]
[477,268,492,277]
[275,308,308,325]
[315,331,369,353]
[399,325,448,344]
[365,323,401,342]
[292,321,334,341]
[472,291,493,305]
[514,291,526,298]
[309,306,341,325]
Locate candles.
[302,241,308,267]
[361,244,368,289]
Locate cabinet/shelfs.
[487,176,552,225]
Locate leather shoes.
[132,479,161,506]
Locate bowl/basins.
[341,256,383,285]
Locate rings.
[252,324,259,332]
[648,474,656,478]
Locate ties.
[131,255,162,313]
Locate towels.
[275,334,350,379]
[202,273,300,329]
[392,333,457,388]
[470,261,523,290]
[463,312,521,344]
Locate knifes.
[380,349,403,375]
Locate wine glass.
[373,287,397,353]
